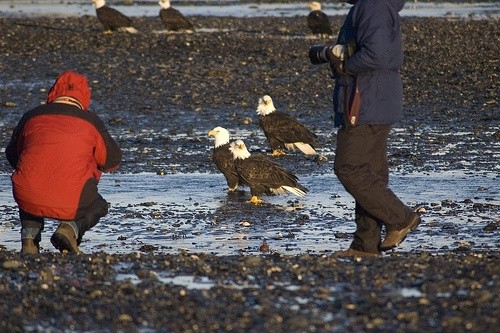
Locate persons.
[5,70,123,253]
[308,0,421,257]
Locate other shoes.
[51,223,84,255]
[21,238,41,254]
[380,213,421,250]
[333,247,379,259]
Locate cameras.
[309,43,356,66]
